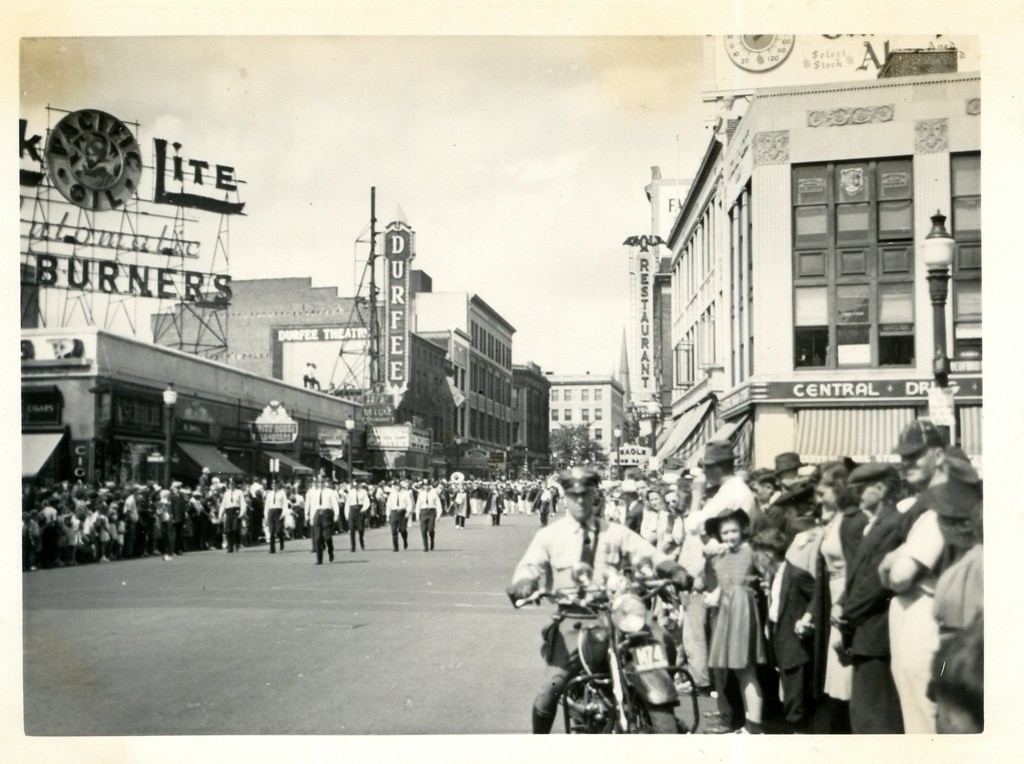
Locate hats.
[847,463,891,487]
[705,508,750,538]
[699,440,739,465]
[772,483,813,506]
[913,476,983,520]
[774,452,806,475]
[892,419,941,454]
[558,467,600,495]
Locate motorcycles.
[513,570,700,735]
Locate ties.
[225,487,433,510]
[577,524,592,598]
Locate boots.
[532,703,557,734]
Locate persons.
[506,469,695,734]
[598,420,984,735]
[22,472,567,571]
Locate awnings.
[686,411,751,467]
[323,455,370,475]
[22,433,63,477]
[657,399,714,461]
[178,443,247,475]
[959,406,984,470]
[795,409,916,464]
[260,450,314,474]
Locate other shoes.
[704,724,730,734]
[703,709,720,718]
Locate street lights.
[920,207,958,390]
[613,425,623,482]
[160,382,179,489]
[344,414,356,488]
[454,436,463,473]
